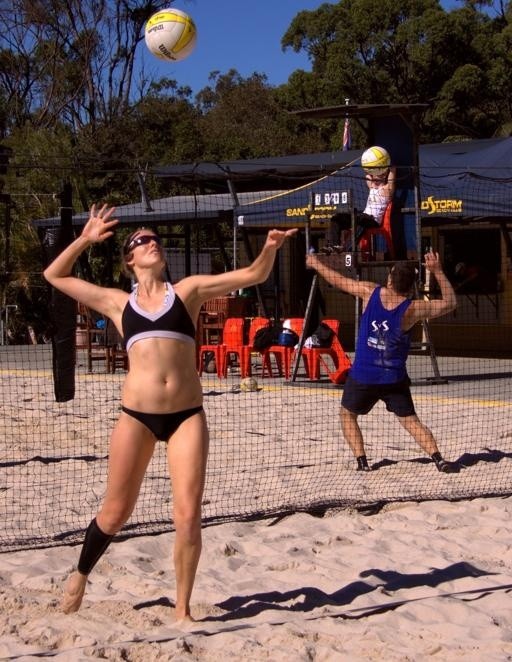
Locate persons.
[42,203,301,622]
[304,244,456,472]
[319,162,395,254]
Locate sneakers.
[355,456,371,471]
[319,244,339,254]
[431,450,450,473]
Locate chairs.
[85,294,354,387]
[343,202,397,261]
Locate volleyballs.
[145,7,196,62]
[362,147,390,175]
[241,377,257,392]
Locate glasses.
[124,235,162,253]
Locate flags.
[339,118,353,154]
